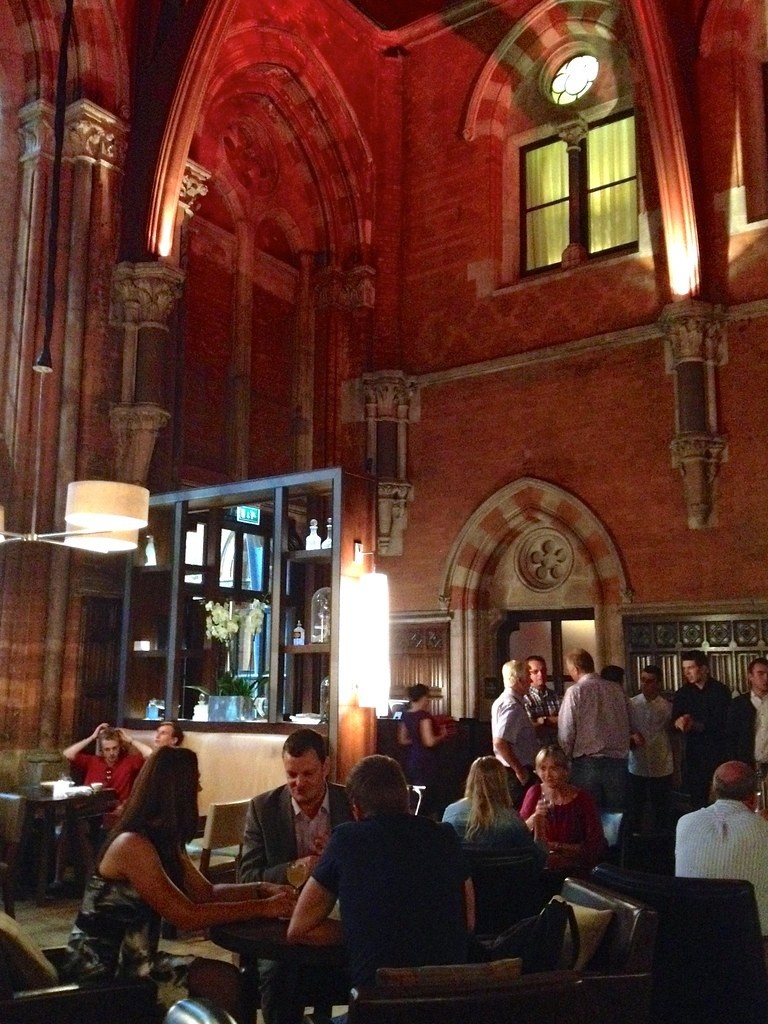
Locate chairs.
[0,767,767,1023]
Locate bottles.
[305,517,332,550]
[294,621,305,645]
[146,705,158,720]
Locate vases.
[204,693,257,722]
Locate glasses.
[529,669,547,675]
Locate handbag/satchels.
[467,896,581,976]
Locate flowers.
[184,604,264,696]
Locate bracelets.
[256,880,262,899]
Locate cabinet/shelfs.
[111,500,220,724]
[268,468,341,725]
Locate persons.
[518,744,608,877]
[238,728,357,1024]
[675,760,768,936]
[398,684,447,825]
[442,757,564,936]
[491,651,766,869]
[48,721,183,892]
[60,747,298,1024]
[287,754,477,1024]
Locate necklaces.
[542,791,562,805]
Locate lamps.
[1,375,150,556]
[33,4,76,377]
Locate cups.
[542,792,552,807]
[53,783,68,800]
[91,784,103,790]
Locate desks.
[24,786,116,894]
[211,916,351,1023]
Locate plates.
[40,781,75,792]
